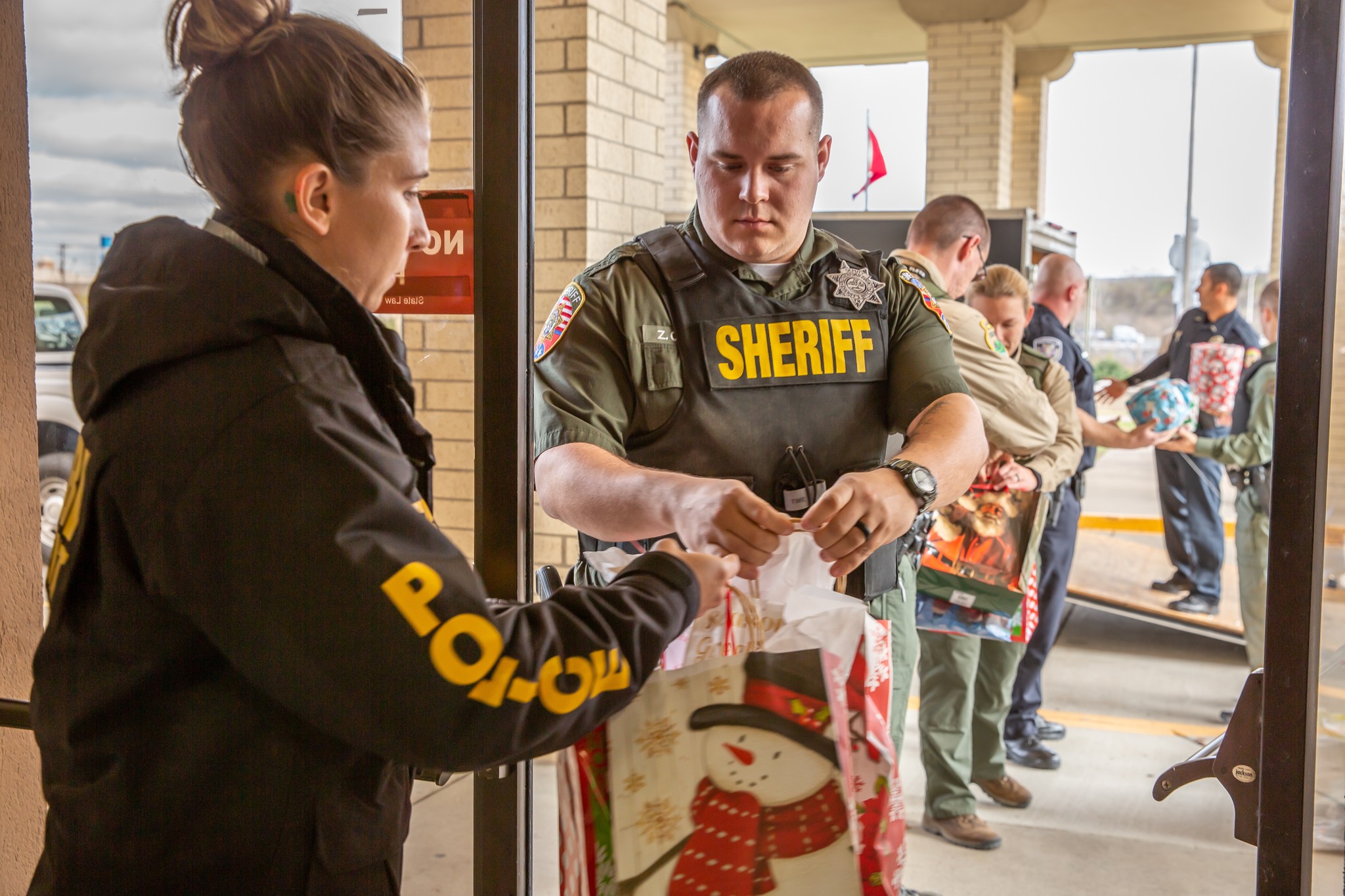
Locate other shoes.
[1152,579,1195,594]
[1168,594,1220,616]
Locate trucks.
[810,205,1077,304]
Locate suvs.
[34,284,88,560]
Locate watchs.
[875,457,938,513]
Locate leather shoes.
[1032,716,1066,740]
[1003,735,1061,771]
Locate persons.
[29,0,740,894]
[876,193,1060,774]
[534,52,986,777]
[1158,275,1282,724]
[1007,251,1181,772]
[921,262,1082,850]
[1093,263,1263,616]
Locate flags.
[852,128,887,197]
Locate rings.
[856,522,871,541]
[1015,474,1020,481]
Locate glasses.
[959,233,987,282]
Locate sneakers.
[921,813,1000,849]
[969,770,1031,806]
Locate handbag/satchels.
[554,584,864,895]
[915,465,1048,620]
[914,560,1042,644]
[743,516,905,896]
